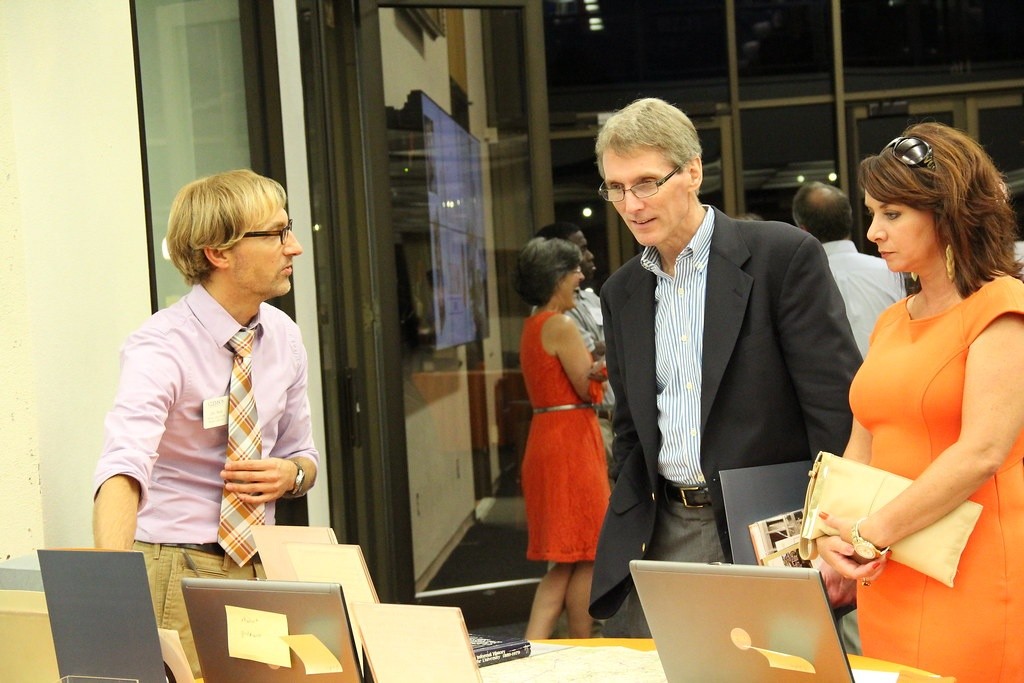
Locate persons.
[530,221,616,572]
[512,237,609,640]
[588,96,865,637]
[791,180,907,361]
[816,120,1024,683]
[93,170,320,679]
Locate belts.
[658,473,713,509]
[136,541,262,562]
[599,411,613,421]
[533,403,593,413]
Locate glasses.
[598,164,682,202]
[561,264,582,274]
[242,219,294,246]
[880,136,937,172]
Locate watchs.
[850,518,891,560]
[287,462,306,496]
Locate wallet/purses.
[798,451,984,589]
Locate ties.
[217,329,265,568]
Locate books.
[468,634,531,667]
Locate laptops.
[628,559,857,683]
[180,577,366,683]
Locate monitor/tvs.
[398,90,490,348]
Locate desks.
[193,639,942,683]
